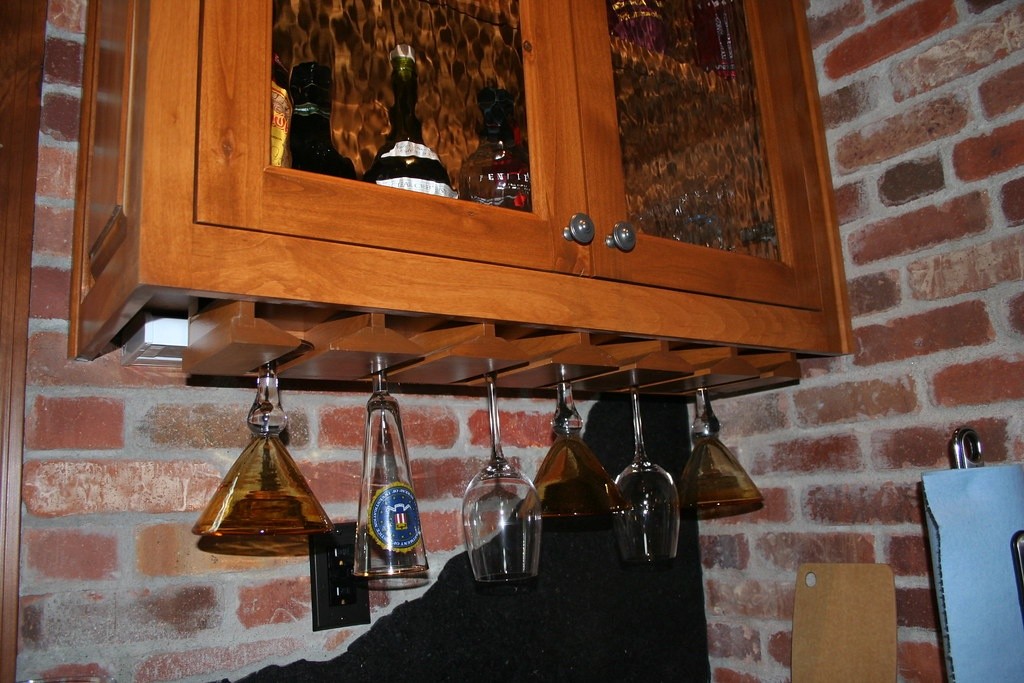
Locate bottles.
[459,87,534,213]
[363,42,462,202]
[295,60,358,178]
[270,48,294,173]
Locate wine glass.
[351,361,431,578]
[607,384,681,563]
[679,388,765,512]
[462,372,542,583]
[530,367,632,524]
[191,338,334,540]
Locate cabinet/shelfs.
[67,0,853,401]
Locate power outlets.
[309,523,371,631]
